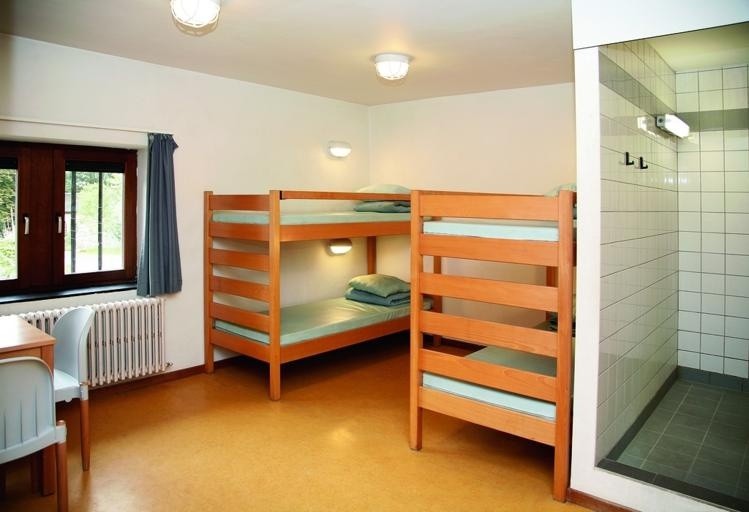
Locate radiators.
[0,295,174,388]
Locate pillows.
[354,183,410,203]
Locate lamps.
[373,52,410,81]
[169,0,222,29]
[327,141,352,158]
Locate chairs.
[0,306,97,512]
